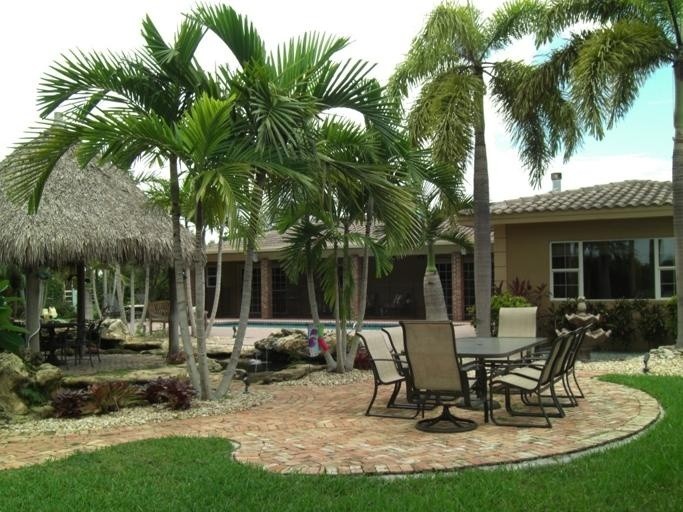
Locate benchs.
[148,299,194,335]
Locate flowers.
[42,306,59,320]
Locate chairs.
[353,306,594,432]
[39,318,104,366]
